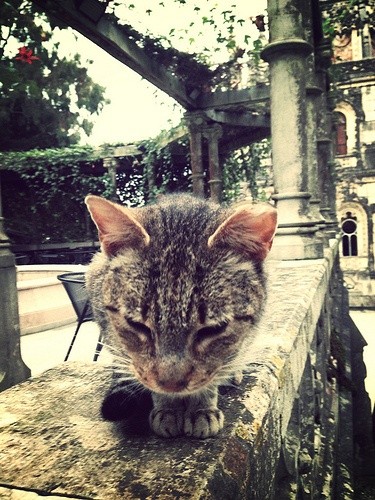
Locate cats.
[82,192,278,441]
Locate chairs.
[57,272,105,361]
